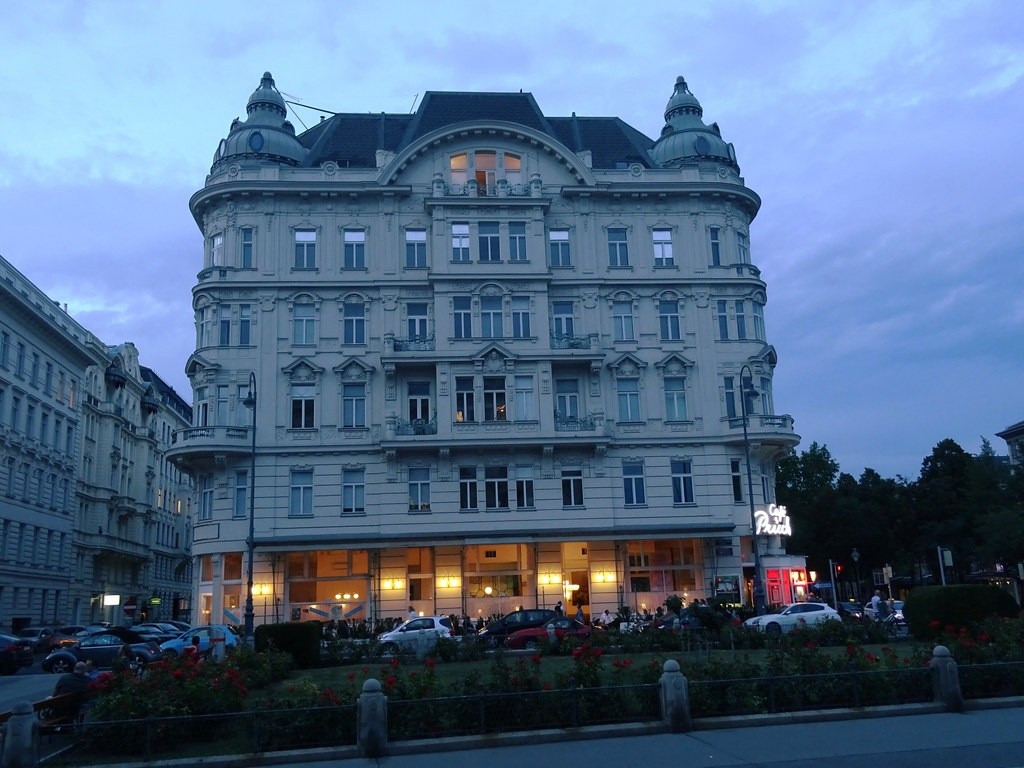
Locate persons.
[576,603,584,623]
[441,605,525,637]
[184,635,200,663]
[635,607,664,629]
[320,616,403,640]
[555,601,565,616]
[408,606,417,620]
[84,656,100,680]
[681,597,783,628]
[599,609,614,629]
[109,643,132,677]
[39,662,92,718]
[808,589,899,634]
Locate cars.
[475,609,559,648]
[863,600,906,625]
[376,616,456,653]
[633,604,742,641]
[42,630,163,674]
[159,626,240,656]
[504,616,609,648]
[742,602,842,637]
[838,602,864,621]
[0,632,35,675]
[16,627,54,653]
[45,621,191,648]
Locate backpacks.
[555,604,563,617]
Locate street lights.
[740,365,767,616]
[243,372,257,653]
[252,582,271,625]
[851,548,862,602]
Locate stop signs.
[123,601,137,616]
